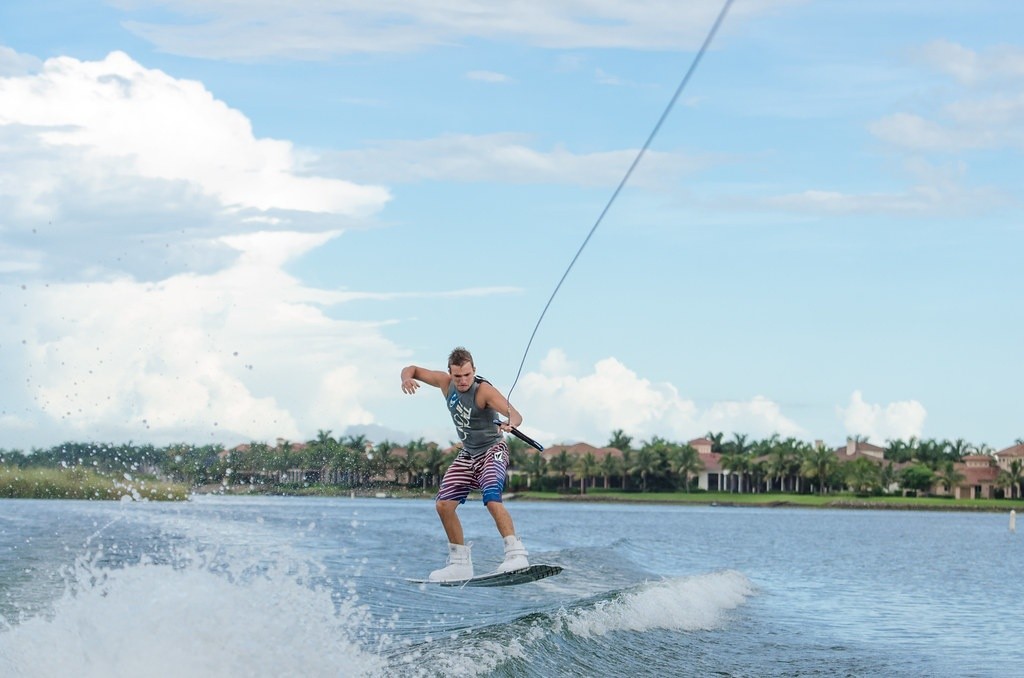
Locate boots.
[498,535,529,572]
[429,541,474,581]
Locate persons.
[400,348,530,579]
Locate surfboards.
[403,563,564,588]
